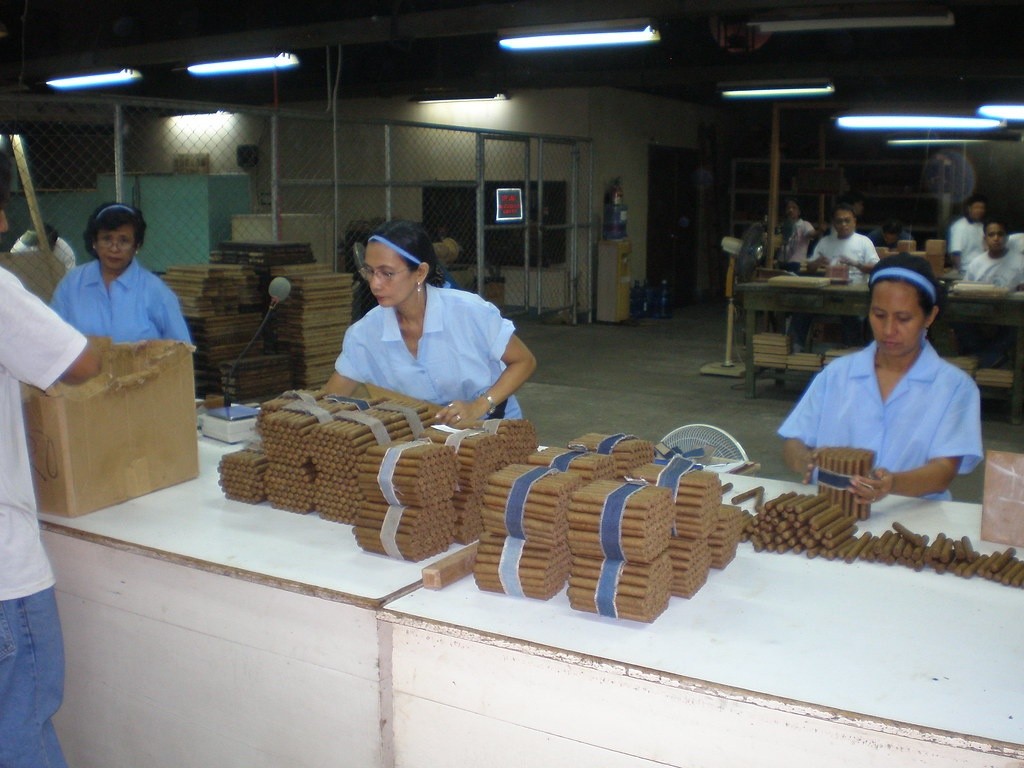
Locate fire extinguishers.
[602,176,625,233]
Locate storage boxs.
[20,335,199,518]
[171,153,209,174]
[980,450,1024,548]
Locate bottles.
[948,329,960,356]
[603,192,628,239]
[630,278,670,317]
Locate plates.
[443,238,459,262]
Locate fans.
[660,424,749,461]
[699,222,767,378]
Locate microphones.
[221,277,291,410]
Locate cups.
[432,242,449,257]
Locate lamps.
[716,78,834,101]
[831,106,1008,131]
[746,4,956,32]
[495,16,661,51]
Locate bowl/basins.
[779,262,800,273]
[825,264,849,278]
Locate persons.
[46,202,193,349]
[319,219,538,428]
[0,147,112,768]
[945,196,1024,367]
[10,223,75,272]
[778,191,913,346]
[776,249,985,501]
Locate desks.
[739,281,1024,424]
[37,429,1024,768]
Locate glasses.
[94,233,137,250]
[359,267,410,288]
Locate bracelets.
[482,392,496,414]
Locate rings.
[456,415,462,421]
[449,403,453,407]
